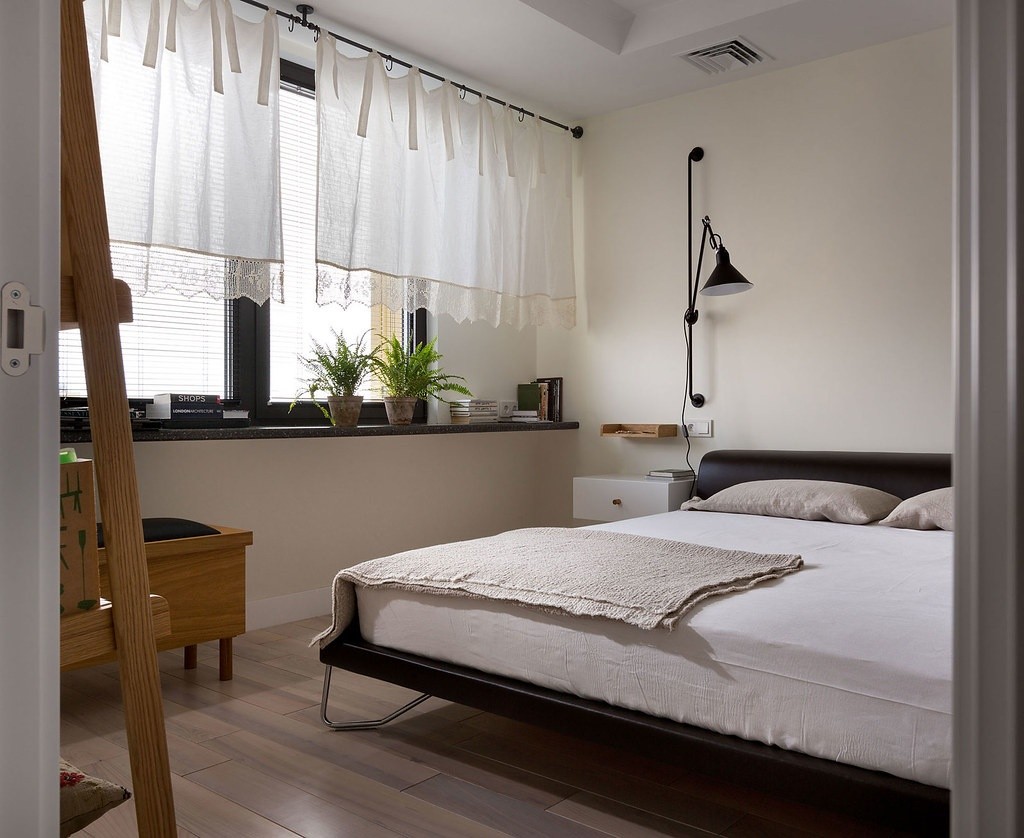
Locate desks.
[97,518,254,681]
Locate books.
[145,393,250,421]
[517,375,563,423]
[450,398,499,424]
[644,468,697,481]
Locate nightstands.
[573,474,698,522]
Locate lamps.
[684,146,753,410]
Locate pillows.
[877,486,953,532]
[60,756,132,838]
[681,479,902,525]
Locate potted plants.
[355,331,475,424]
[287,327,394,427]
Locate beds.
[303,450,952,838]
